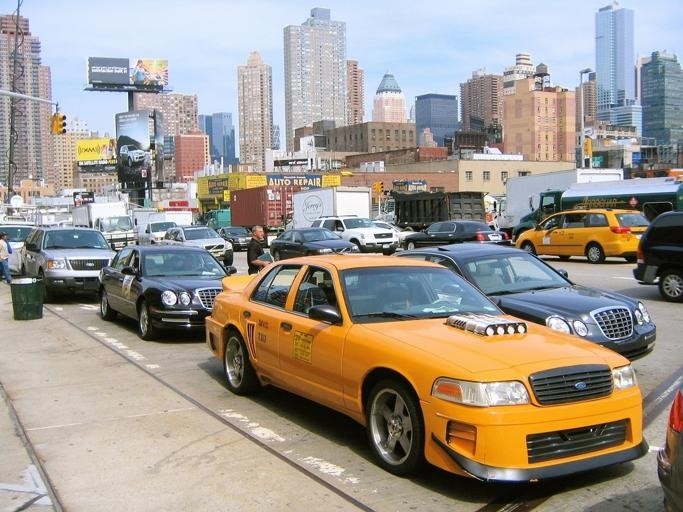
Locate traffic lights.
[56,113,67,135]
[50,114,57,134]
[372,181,378,194]
[378,181,383,193]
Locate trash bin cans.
[265,227,285,248]
[10,278,43,320]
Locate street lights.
[578,68,592,168]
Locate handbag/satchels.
[6,240,12,254]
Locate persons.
[0,232,13,283]
[171,255,195,271]
[131,60,150,85]
[247,225,271,276]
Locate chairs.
[473,261,506,291]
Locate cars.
[489,212,498,231]
[218,225,254,251]
[202,252,647,489]
[96,244,237,341]
[370,221,418,248]
[654,388,682,512]
[402,220,511,250]
[515,206,649,265]
[391,243,656,365]
[268,227,360,263]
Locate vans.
[0,221,37,278]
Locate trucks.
[508,176,682,243]
[493,167,624,239]
[374,190,489,232]
[280,185,372,230]
[205,183,301,234]
[0,202,194,251]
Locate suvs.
[119,143,145,167]
[162,225,234,265]
[632,210,682,302]
[18,225,119,303]
[310,215,401,256]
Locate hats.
[0,232,9,236]
